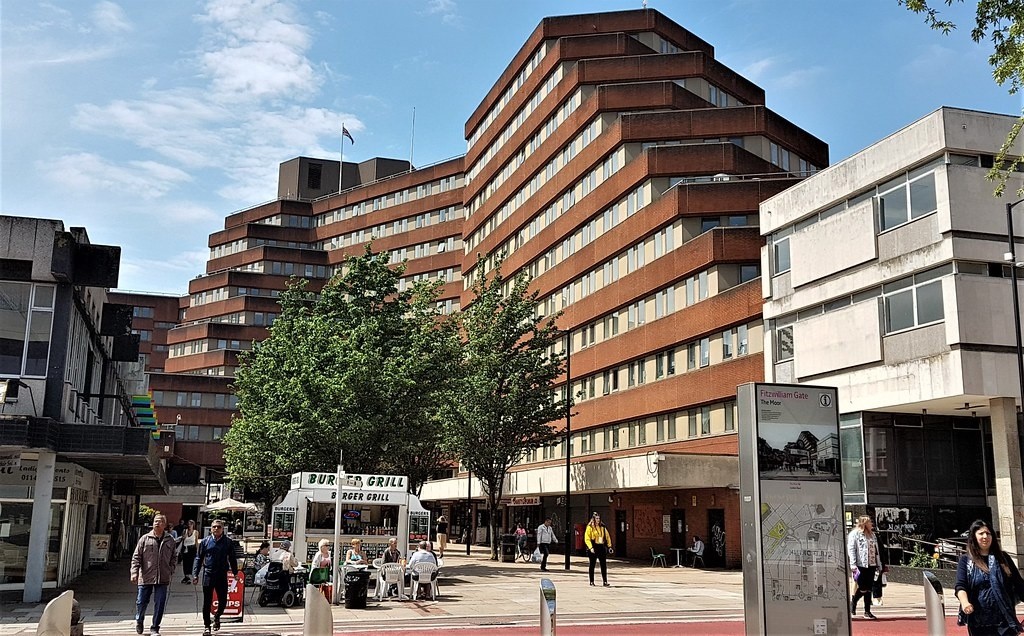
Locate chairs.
[375,563,404,603]
[372,557,406,595]
[648,544,669,569]
[420,557,444,597]
[250,564,262,601]
[336,565,361,606]
[409,561,440,602]
[691,543,707,567]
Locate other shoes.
[603,582,610,586]
[540,566,549,571]
[136,620,143,634]
[850,603,856,615]
[150,626,161,636]
[213,619,220,631]
[864,612,876,619]
[181,578,186,583]
[204,627,211,636]
[186,578,191,585]
[440,553,444,558]
[589,582,595,587]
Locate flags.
[343,128,354,145]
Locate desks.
[339,564,370,599]
[670,547,687,568]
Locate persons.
[380,536,401,596]
[846,515,883,621]
[536,517,559,572]
[954,519,1024,636]
[129,514,176,635]
[192,519,241,636]
[585,513,614,587]
[435,516,448,559]
[679,535,704,567]
[872,568,888,605]
[309,539,333,582]
[513,523,526,557]
[344,540,369,565]
[174,519,188,564]
[252,542,271,574]
[181,519,199,585]
[165,523,178,538]
[272,540,298,574]
[408,540,439,598]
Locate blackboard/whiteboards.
[305,541,390,564]
[241,567,259,586]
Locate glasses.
[212,525,222,530]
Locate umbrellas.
[198,498,257,532]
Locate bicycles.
[514,537,532,562]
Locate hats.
[280,541,290,548]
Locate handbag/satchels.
[532,546,542,563]
[255,562,270,586]
[882,573,887,587]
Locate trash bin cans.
[344,570,371,610]
[499,534,517,563]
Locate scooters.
[257,562,303,608]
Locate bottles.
[399,556,407,567]
[874,571,880,581]
[341,523,396,536]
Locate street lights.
[552,328,570,570]
